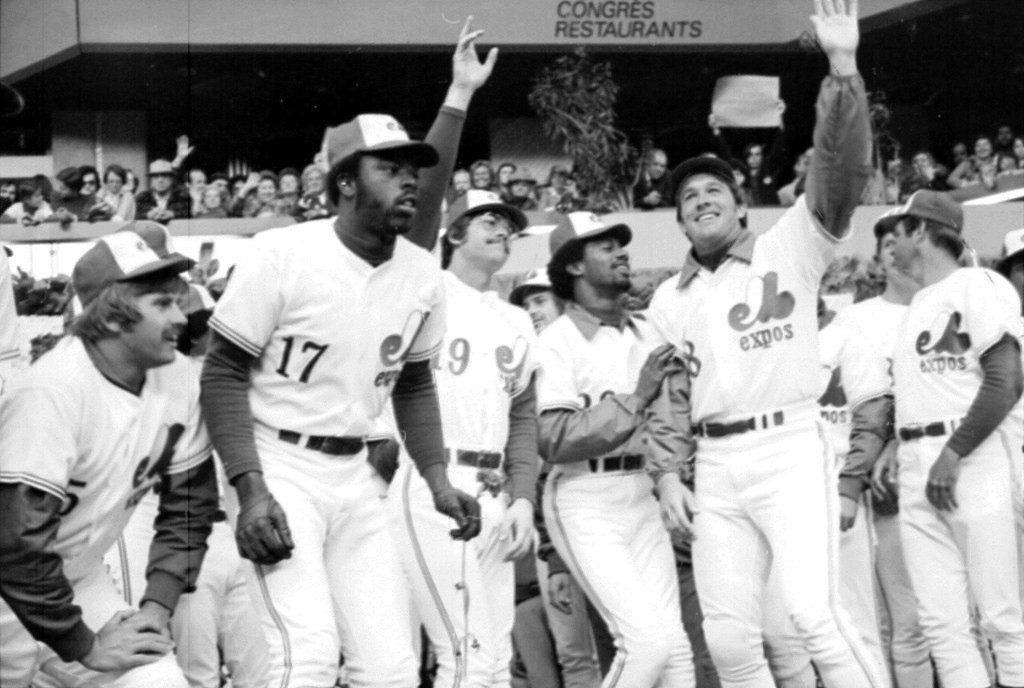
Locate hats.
[446,189,529,231]
[552,164,568,173]
[549,210,632,257]
[508,166,536,182]
[320,113,440,173]
[873,205,904,237]
[57,166,80,182]
[880,189,963,231]
[149,159,172,173]
[73,220,194,307]
[670,152,736,194]
[183,283,215,315]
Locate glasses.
[82,180,95,186]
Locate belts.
[279,428,365,455]
[697,411,783,437]
[443,447,502,468]
[589,453,646,472]
[899,417,966,441]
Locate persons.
[202,114,485,688]
[1,0,1024,688]
